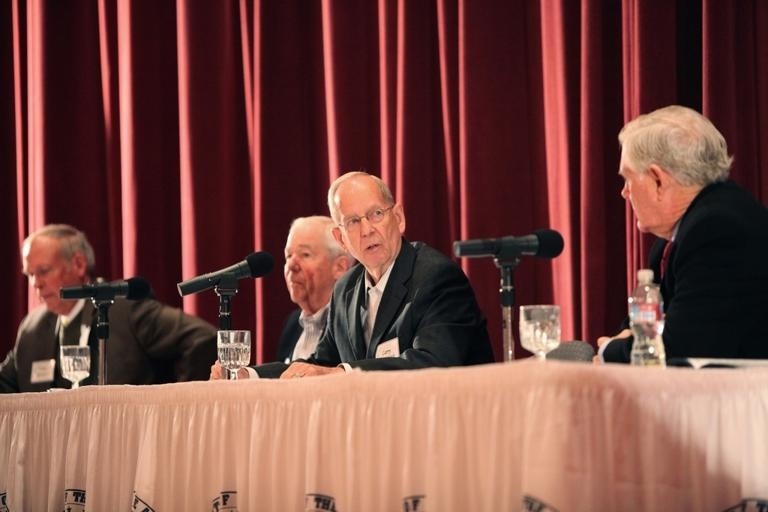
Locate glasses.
[338,206,396,231]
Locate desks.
[2,362,768,511]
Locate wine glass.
[218,331,251,379]
[59,345,91,389]
[519,304,563,361]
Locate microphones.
[60,276,150,300]
[177,251,275,297]
[452,228,564,259]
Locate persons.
[593,105,768,366]
[209,172,495,381]
[0,223,218,394]
[271,215,356,362]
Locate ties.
[660,240,673,284]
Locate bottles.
[629,269,666,367]
